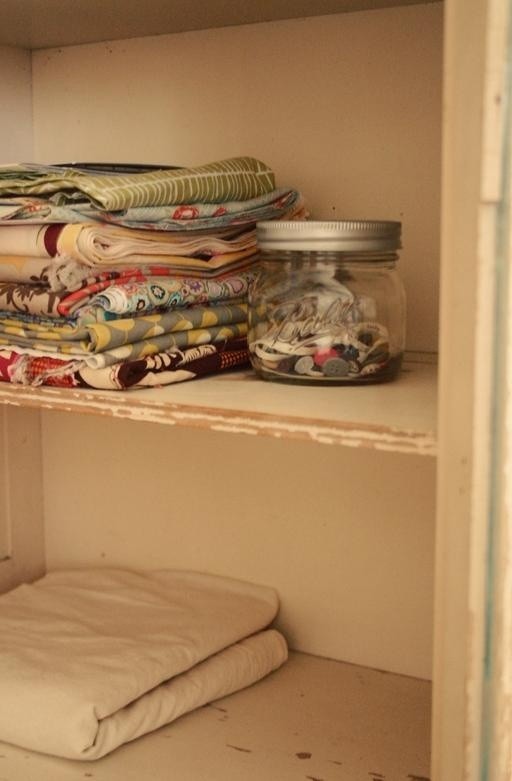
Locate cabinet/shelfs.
[0,0,510,780]
